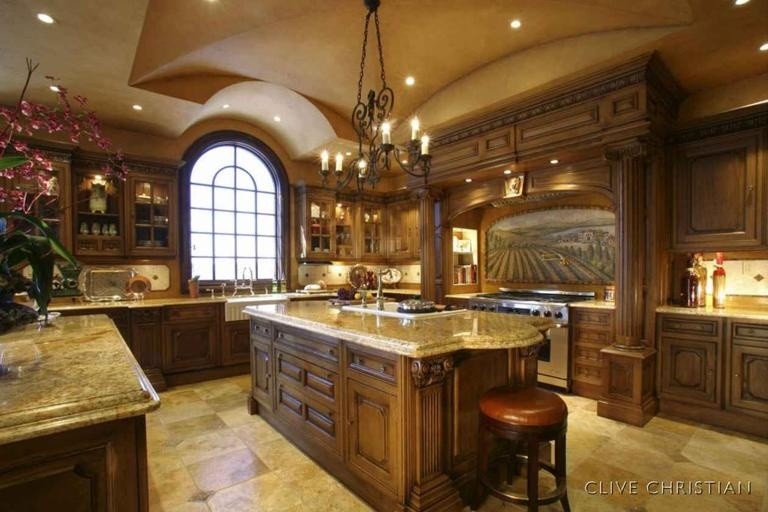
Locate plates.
[153,215,168,223]
[135,239,165,247]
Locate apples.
[355,293,362,300]
[365,292,373,300]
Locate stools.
[473,386,571,511]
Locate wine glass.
[79,222,118,237]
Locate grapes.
[337,288,354,300]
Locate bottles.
[454,264,477,284]
[681,252,726,308]
[271,275,287,293]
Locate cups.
[188,280,200,298]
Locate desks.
[1,415,148,512]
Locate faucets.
[241,267,254,288]
[376,268,394,299]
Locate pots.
[400,295,436,311]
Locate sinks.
[226,294,289,323]
[342,302,464,317]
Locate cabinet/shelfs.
[570,307,615,401]
[292,180,422,263]
[249,316,539,512]
[667,103,767,253]
[1,135,186,258]
[110,304,251,375]
[725,319,768,438]
[656,314,724,428]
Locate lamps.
[317,0,433,193]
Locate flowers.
[1,60,128,324]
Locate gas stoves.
[468,291,595,325]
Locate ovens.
[535,326,572,392]
